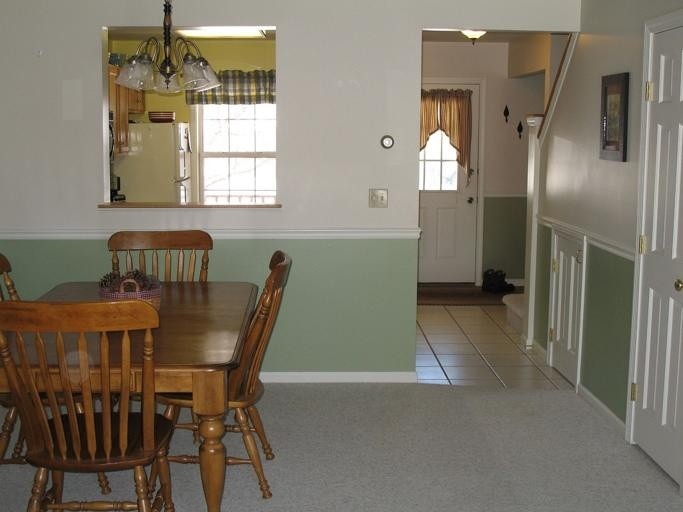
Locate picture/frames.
[599,72,629,162]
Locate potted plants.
[96,269,162,314]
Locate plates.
[147,111,175,123]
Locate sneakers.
[483,268,516,293]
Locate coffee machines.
[110,176,126,201]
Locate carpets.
[417,285,502,305]
[0,382,681,511]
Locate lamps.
[460,29,487,45]
[114,0,223,94]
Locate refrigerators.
[113,121,192,202]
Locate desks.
[3,280,259,510]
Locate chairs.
[153,250,292,500]
[108,232,214,415]
[1,253,121,495]
[1,298,177,512]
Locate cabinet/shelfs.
[109,65,145,154]
